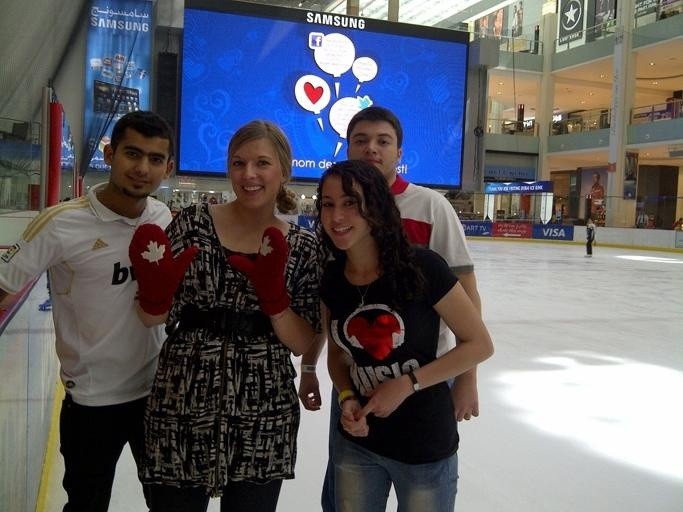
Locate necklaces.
[348,267,384,306]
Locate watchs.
[407,370,420,393]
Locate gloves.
[227,224,294,317]
[127,221,200,317]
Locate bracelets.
[301,364,317,374]
[336,389,358,408]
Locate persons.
[589,172,604,200]
[584,217,596,258]
[127,120,326,512]
[511,1,523,37]
[0,110,173,512]
[316,160,495,512]
[670,218,683,231]
[296,106,480,512]
[635,210,649,229]
